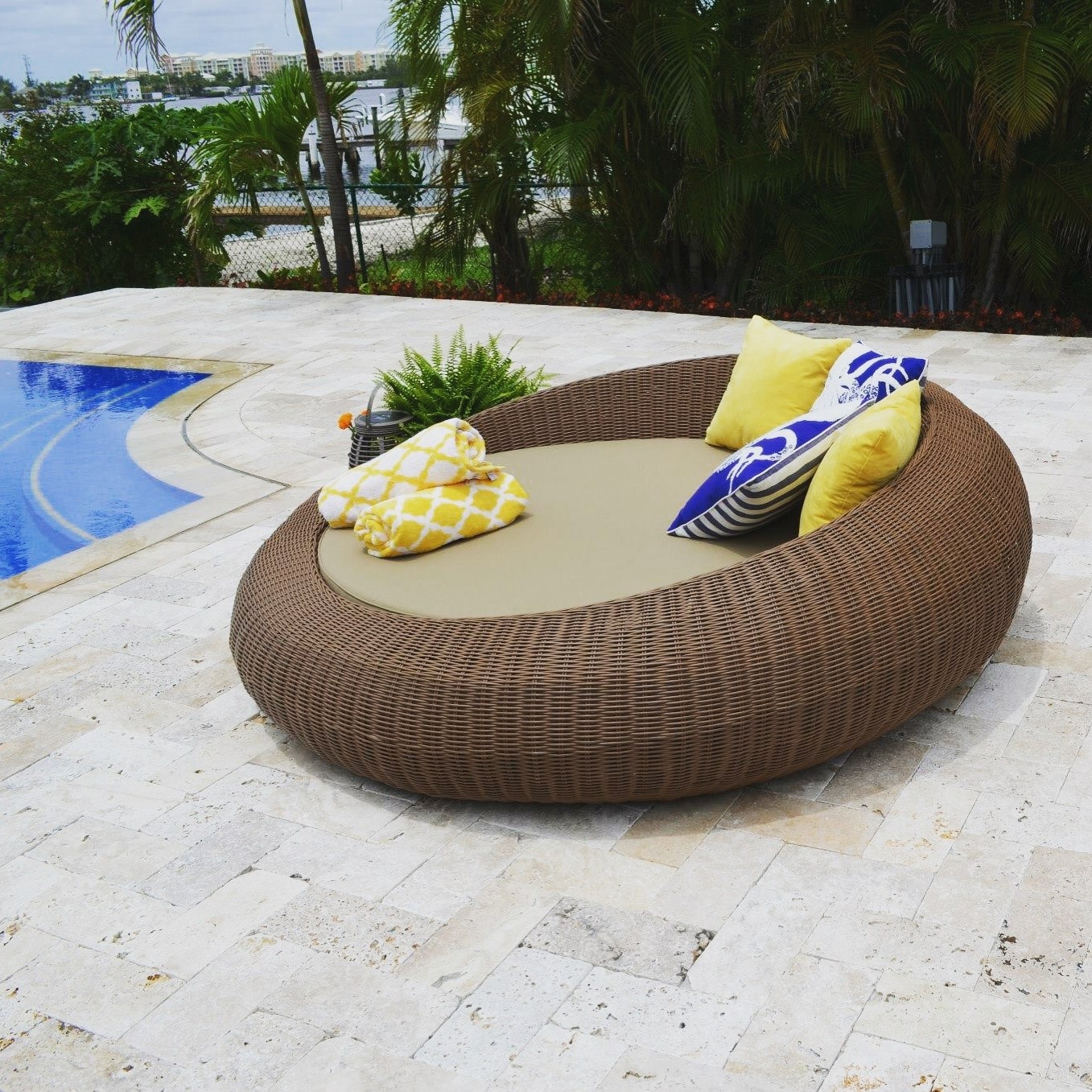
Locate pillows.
[808,338,925,413]
[666,396,876,538]
[702,316,858,450]
[800,377,924,538]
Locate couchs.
[225,351,1036,807]
